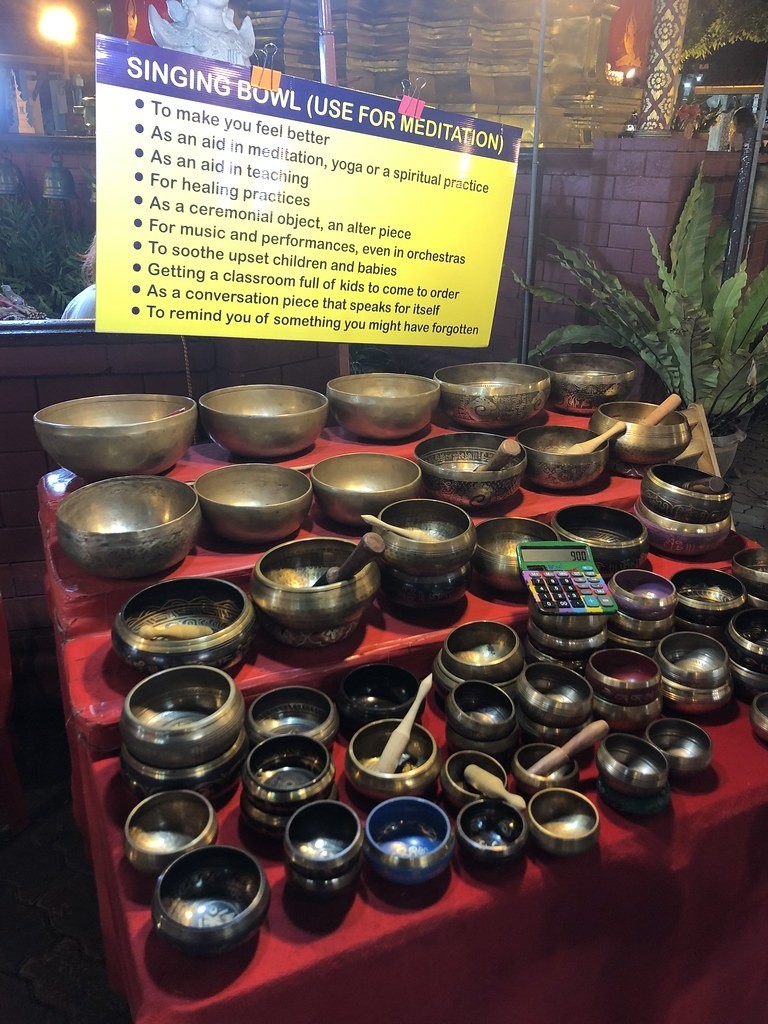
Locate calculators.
[516,540,619,615]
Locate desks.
[35,403,768,1024]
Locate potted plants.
[505,158,768,479]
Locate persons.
[62,237,94,319]
[730,107,755,154]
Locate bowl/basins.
[250,536,381,648]
[199,384,327,457]
[433,362,550,430]
[589,401,691,477]
[55,476,202,578]
[551,503,648,578]
[732,547,768,601]
[517,424,609,493]
[327,373,441,438]
[111,578,255,677]
[370,499,470,605]
[415,432,527,508]
[194,463,312,544]
[431,568,768,863]
[472,517,562,592]
[310,452,422,526]
[538,353,634,414]
[632,464,733,553]
[118,666,455,946]
[32,393,197,482]
